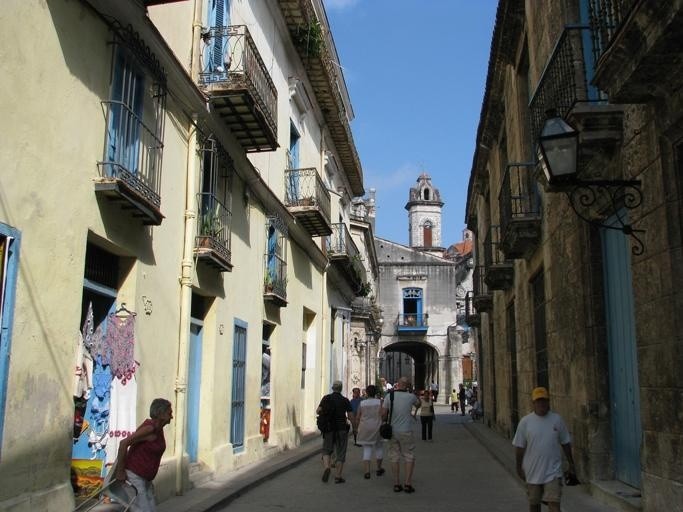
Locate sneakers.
[321,468,346,485]
[364,468,386,479]
[393,484,415,494]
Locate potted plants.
[196,202,220,247]
[263,270,276,293]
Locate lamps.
[530,100,647,256]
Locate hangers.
[108,302,137,320]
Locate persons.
[512,387,577,512]
[117,399,173,512]
[449,381,482,420]
[316,378,441,492]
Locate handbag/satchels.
[379,423,393,440]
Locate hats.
[531,387,549,403]
[333,381,342,388]
